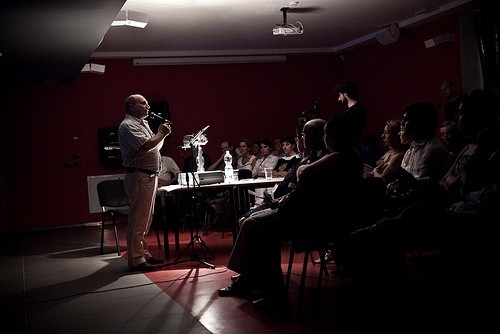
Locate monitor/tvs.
[146,101,171,128]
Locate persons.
[157,155,181,187]
[182,77,500,334]
[119,93,171,272]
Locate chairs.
[282,236,350,303]
[96,178,163,255]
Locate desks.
[156,176,287,261]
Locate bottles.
[224,151,233,180]
[196,151,205,171]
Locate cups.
[224,174,232,183]
[233,170,239,181]
[264,168,272,180]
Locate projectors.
[272,21,304,37]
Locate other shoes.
[219,274,289,312]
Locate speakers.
[97,126,122,168]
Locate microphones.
[150,111,170,122]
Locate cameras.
[299,98,320,123]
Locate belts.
[127,166,161,176]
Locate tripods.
[159,125,215,265]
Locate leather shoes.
[145,258,164,264]
[131,261,161,270]
